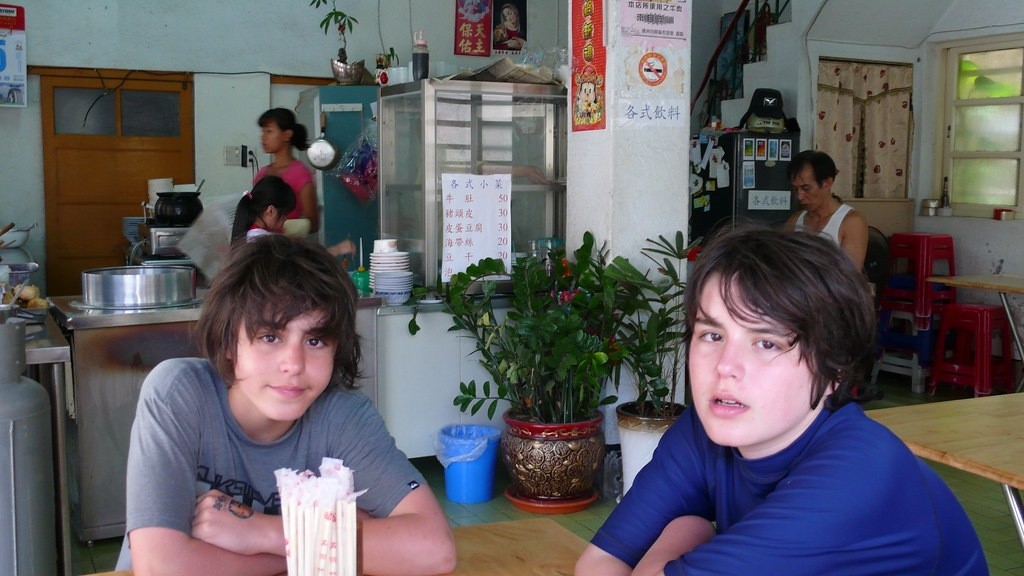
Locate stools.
[928,302,1015,398]
[870,232,958,394]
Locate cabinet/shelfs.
[377,79,567,304]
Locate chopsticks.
[273,457,357,576]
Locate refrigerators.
[299,85,380,278]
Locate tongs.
[11,306,45,321]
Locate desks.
[925,273,1024,393]
[86,515,587,576]
[865,393,1024,556]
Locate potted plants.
[376,48,409,86]
[406,230,702,503]
[309,0,365,86]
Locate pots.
[307,113,339,171]
[154,192,203,228]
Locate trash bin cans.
[436,424,501,504]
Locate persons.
[575,217,992,576]
[114,231,456,576]
[254,108,320,234]
[230,176,356,258]
[784,150,868,273]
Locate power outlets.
[247,145,257,167]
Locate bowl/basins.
[122,217,146,246]
[9,263,40,287]
[374,239,398,253]
[374,271,413,306]
[0,228,30,248]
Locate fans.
[847,226,893,403]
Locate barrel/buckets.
[0,304,20,324]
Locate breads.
[11,284,49,309]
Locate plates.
[369,252,410,289]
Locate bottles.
[412,30,429,80]
[1,265,11,283]
[352,267,369,297]
[940,176,950,208]
